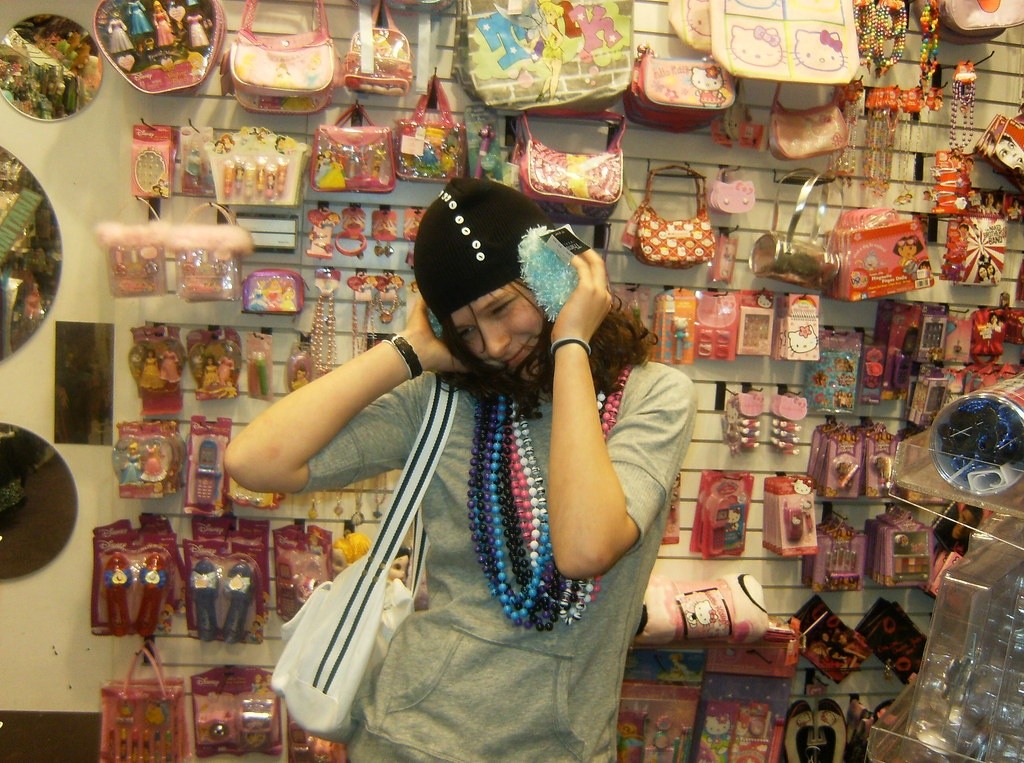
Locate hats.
[414,178,556,316]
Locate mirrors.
[0,13,104,121]
[0,146,63,361]
[54,321,113,447]
[0,420,79,582]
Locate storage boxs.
[616,632,799,763]
[876,301,972,425]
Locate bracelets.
[827,0,978,201]
[382,329,424,378]
[551,338,592,358]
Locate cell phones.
[194,439,220,507]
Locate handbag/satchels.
[173,203,247,300]
[343,0,413,97]
[312,104,396,193]
[392,75,468,183]
[523,110,624,204]
[708,172,755,214]
[102,195,168,297]
[750,168,845,290]
[940,0,1024,36]
[634,165,717,270]
[624,46,738,129]
[100,640,191,763]
[270,374,459,742]
[828,210,935,301]
[769,80,850,160]
[230,0,334,115]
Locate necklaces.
[464,363,633,627]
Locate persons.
[127,0,154,35]
[223,177,699,763]
[151,0,175,47]
[108,11,134,54]
[184,0,210,49]
